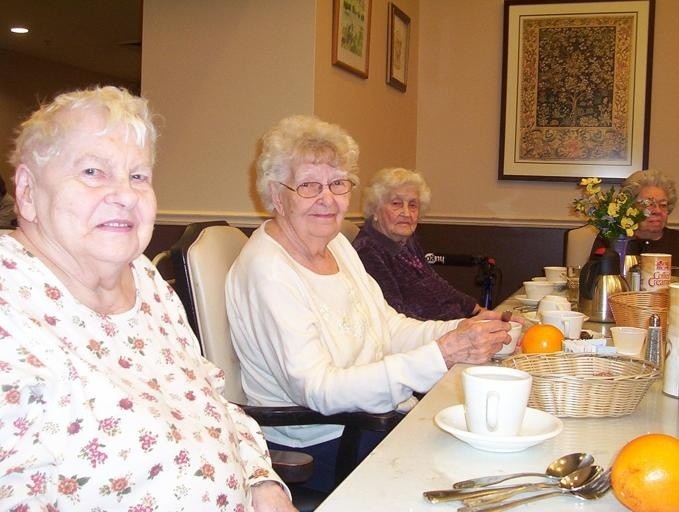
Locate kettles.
[577,247,631,323]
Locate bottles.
[644,314,661,367]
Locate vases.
[605,230,631,277]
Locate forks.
[479,467,612,512]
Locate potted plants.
[572,176,653,239]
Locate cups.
[536,295,572,318]
[522,281,555,299]
[474,320,523,356]
[541,310,585,340]
[462,365,533,436]
[543,266,567,281]
[610,326,647,356]
[567,265,583,303]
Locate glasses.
[607,293,670,343]
[277,179,355,199]
[642,203,675,212]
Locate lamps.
[9,26,26,32]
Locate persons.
[591,169,678,274]
[348,165,488,322]
[228,113,523,495]
[0,90,304,512]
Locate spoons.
[453,453,595,491]
[421,465,604,506]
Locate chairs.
[175,220,357,503]
[269,448,315,508]
[151,247,174,287]
[338,216,359,240]
[418,252,499,311]
[564,217,600,271]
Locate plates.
[523,310,591,323]
[432,404,566,454]
[513,294,540,305]
[531,277,547,281]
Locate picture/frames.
[387,3,411,93]
[329,0,374,81]
[498,0,655,186]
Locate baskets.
[502,353,664,418]
